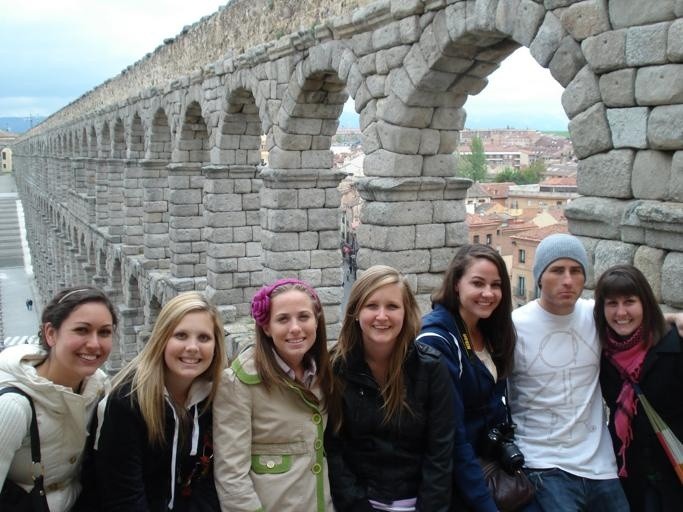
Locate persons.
[341,240,356,281]
[80,291,229,512]
[0,284,119,512]
[29,299,32,309]
[26,299,29,310]
[505,232,683,511]
[212,279,335,512]
[323,265,457,512]
[415,244,518,512]
[593,265,683,511]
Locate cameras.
[477,423,525,476]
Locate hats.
[531,233,589,289]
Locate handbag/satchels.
[0,386,51,512]
[663,431,683,484]
[475,451,537,511]
